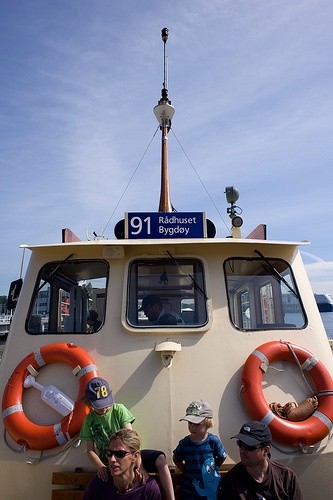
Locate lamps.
[232,216,242,227]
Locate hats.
[230,421,272,446]
[179,400,213,424]
[86,377,113,409]
[138,294,160,311]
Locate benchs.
[51,464,238,500]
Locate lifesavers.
[239,340,332,446]
[1,342,99,450]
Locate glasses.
[236,440,263,451]
[106,450,130,458]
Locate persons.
[138,294,186,325]
[79,377,175,500]
[82,430,161,500]
[217,421,303,500]
[172,400,227,500]
[86,310,102,333]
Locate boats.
[0,27,332,498]
